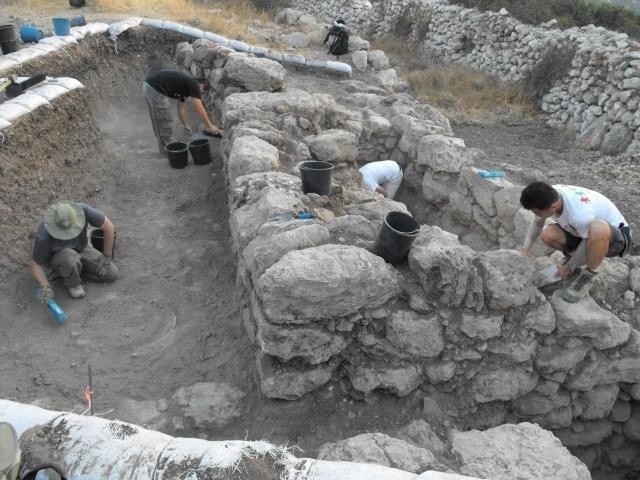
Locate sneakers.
[564,268,598,303]
[69,285,86,299]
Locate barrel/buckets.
[298,161,334,197]
[0,24,18,55]
[375,211,420,265]
[20,24,43,43]
[52,17,71,36]
[189,139,212,166]
[165,141,188,169]
[69,0,86,7]
[71,15,86,28]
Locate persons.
[518,181,633,303]
[143,70,221,152]
[31,200,118,303]
[358,159,403,198]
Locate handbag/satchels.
[324,28,348,55]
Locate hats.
[44,200,86,240]
